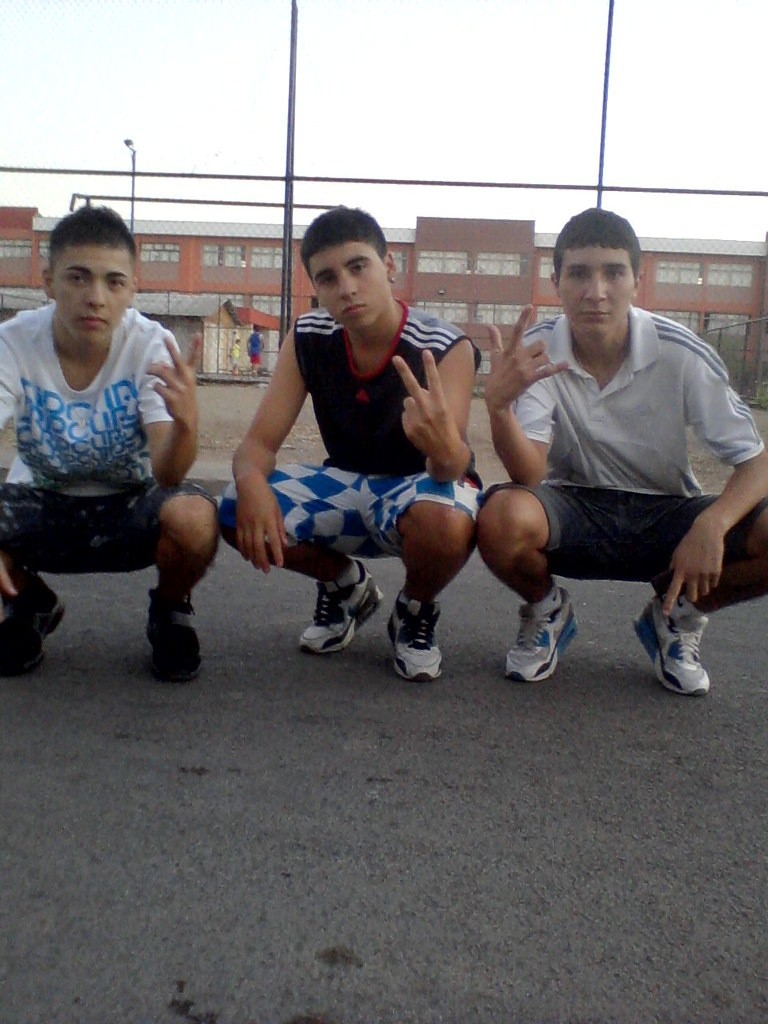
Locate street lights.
[124,139,136,235]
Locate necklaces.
[573,341,613,389]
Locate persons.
[246,326,265,371]
[477,209,768,696]
[229,339,241,375]
[218,209,483,681]
[0,212,220,682]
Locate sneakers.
[387,590,443,681]
[504,588,578,681]
[635,594,710,695]
[146,588,202,682]
[0,572,64,676]
[299,559,385,653]
